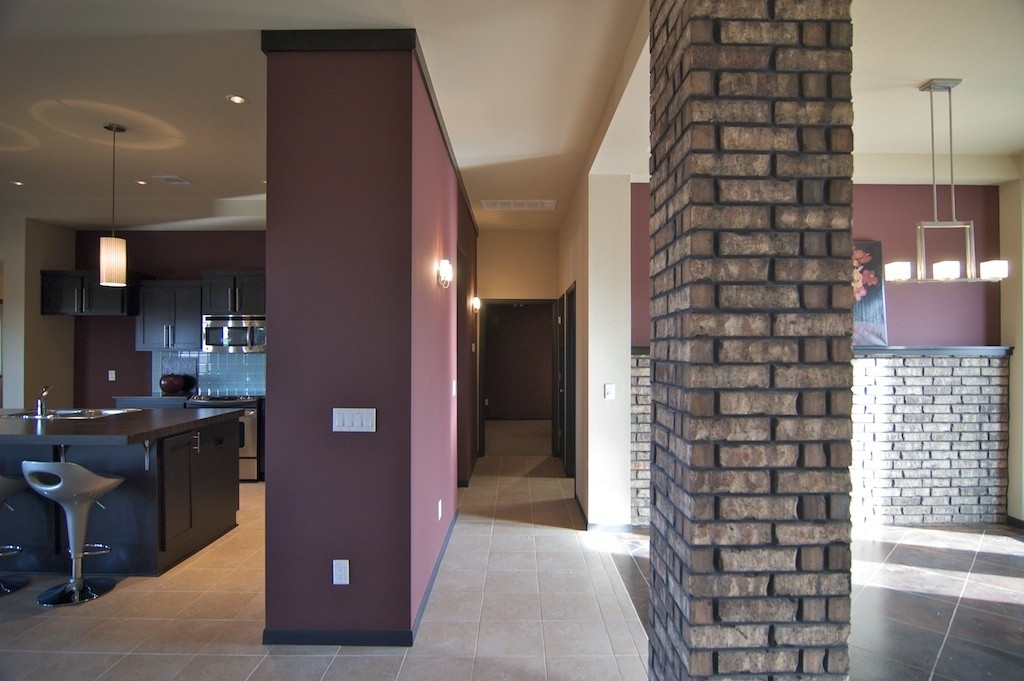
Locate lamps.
[881,72,1010,284]
[437,258,454,289]
[472,296,481,315]
[97,122,128,288]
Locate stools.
[21,458,128,607]
[0,473,30,600]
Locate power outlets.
[333,559,350,585]
[108,370,116,381]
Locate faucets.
[36,383,56,418]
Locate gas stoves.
[186,394,259,408]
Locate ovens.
[239,408,257,480]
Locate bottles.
[160,373,184,396]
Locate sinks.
[55,411,125,419]
[6,411,82,416]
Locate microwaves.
[202,314,267,353]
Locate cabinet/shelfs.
[135,278,203,352]
[202,270,266,315]
[36,265,137,317]
